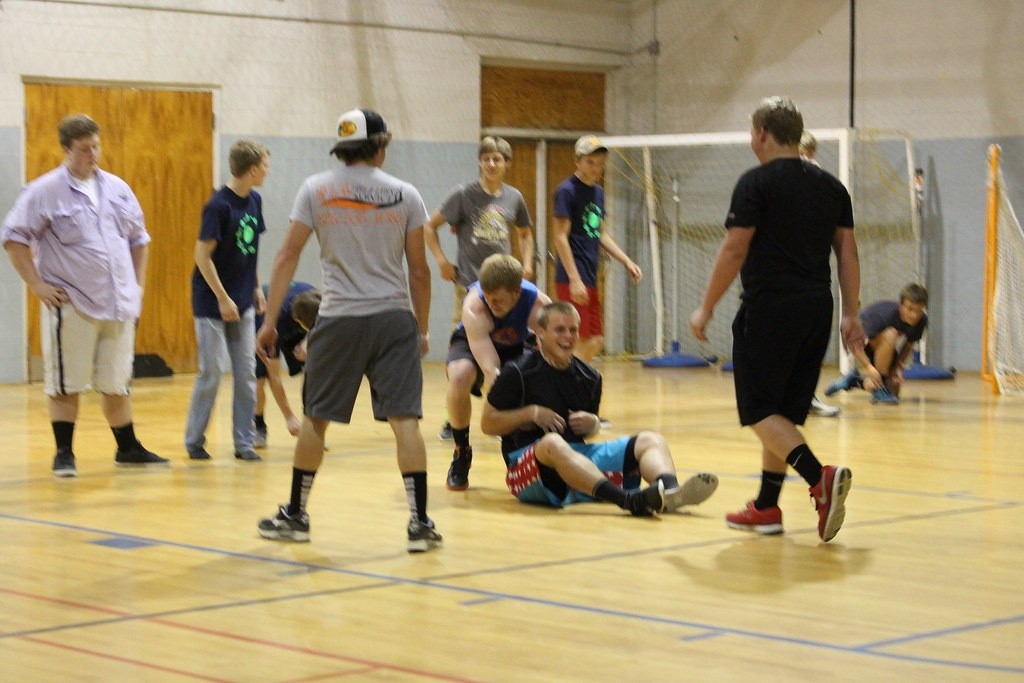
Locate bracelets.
[420,332,431,337]
[533,403,540,423]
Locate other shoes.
[234,450,265,461]
[440,422,455,439]
[189,447,212,460]
[254,428,269,450]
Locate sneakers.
[823,369,860,397]
[724,499,785,535]
[870,385,900,405]
[255,505,312,540]
[628,480,667,519]
[112,441,171,467]
[445,445,475,491]
[660,472,719,513]
[404,516,444,552]
[52,449,79,477]
[810,394,840,416]
[807,465,852,542]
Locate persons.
[183,138,324,462]
[258,108,445,553]
[689,95,866,542]
[445,254,552,491]
[4,114,169,478]
[482,299,718,518]
[825,282,930,405]
[552,134,643,367]
[425,136,533,441]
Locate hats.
[575,135,608,155]
[328,110,389,155]
